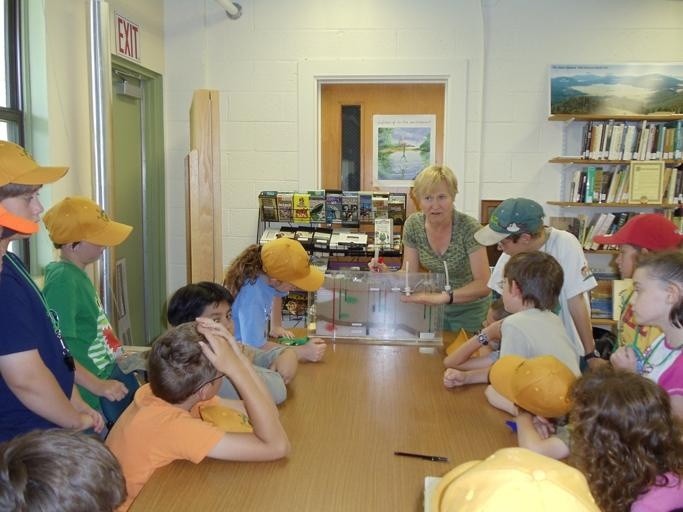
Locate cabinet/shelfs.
[544,113,682,323]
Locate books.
[570,119,683,250]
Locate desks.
[127,333,579,512]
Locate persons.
[403,167,493,333]
[440,193,598,461]
[577,211,681,512]
[0,139,134,437]
[0,426,128,512]
[220,237,328,364]
[100,283,296,509]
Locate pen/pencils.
[394,452,448,461]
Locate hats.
[487,352,578,419]
[593,212,683,252]
[472,197,546,247]
[0,139,68,190]
[1,204,40,236]
[44,196,134,249]
[261,237,325,294]
[446,327,479,359]
